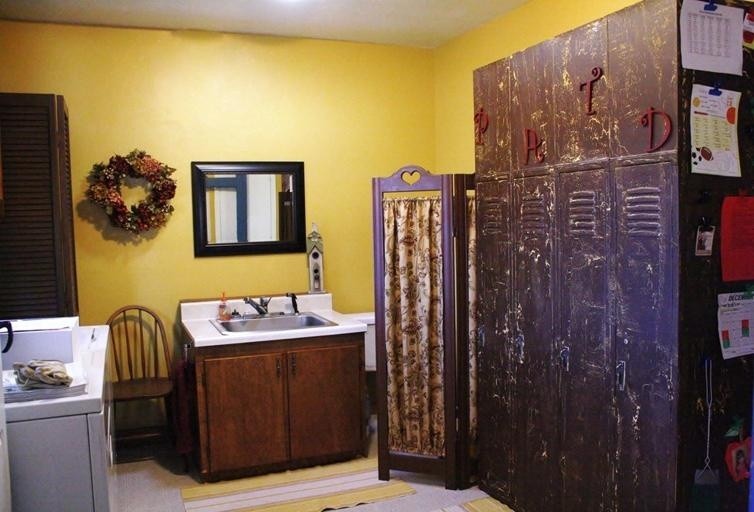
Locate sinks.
[208,311,339,335]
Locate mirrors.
[191,160,308,256]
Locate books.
[2,362,89,404]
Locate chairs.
[105,306,175,463]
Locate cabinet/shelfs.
[179,323,371,485]
[470,1,754,512]
[8,377,114,512]
[1,92,80,320]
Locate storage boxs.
[0,315,81,370]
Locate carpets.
[179,445,418,511]
[441,495,515,512]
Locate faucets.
[242,295,274,313]
